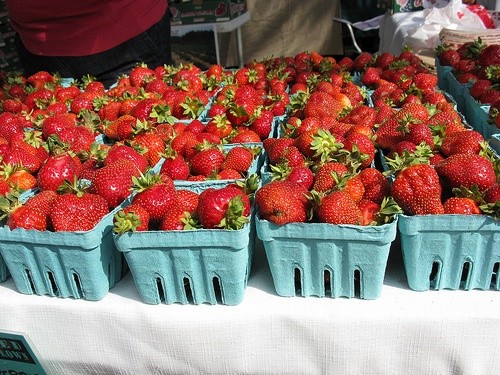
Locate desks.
[171,14,250,69]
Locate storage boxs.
[179,0,247,24]
[0,54,500,306]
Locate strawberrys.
[0,34,500,237]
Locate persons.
[5,0,171,89]
